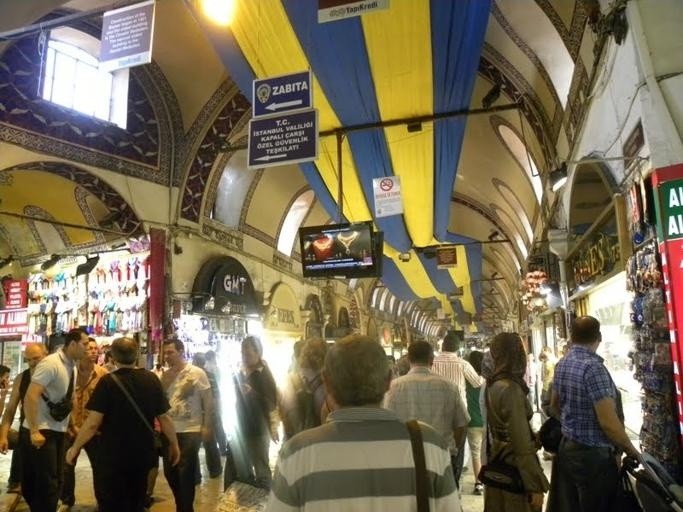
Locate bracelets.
[623,442,633,452]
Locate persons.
[537,350,556,410]
[481,330,552,512]
[543,314,644,512]
[525,353,539,411]
[1,322,496,512]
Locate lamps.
[480,79,512,111]
[0,246,133,276]
[169,292,214,311]
[487,231,500,308]
[181,295,234,316]
[547,153,650,194]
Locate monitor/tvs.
[328,230,384,279]
[298,220,378,279]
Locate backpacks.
[285,370,323,435]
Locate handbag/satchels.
[539,417,562,453]
[41,370,73,421]
[152,431,166,455]
[478,459,528,494]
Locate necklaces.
[312,236,334,253]
[337,230,358,256]
